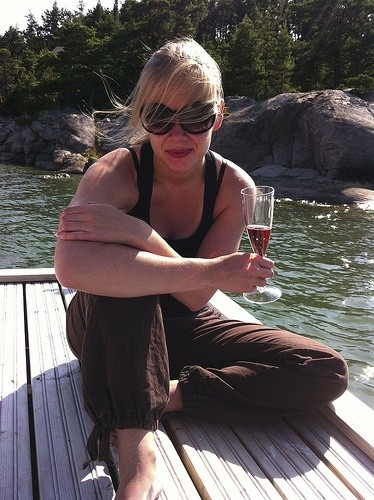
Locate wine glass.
[241,186,282,304]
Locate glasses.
[139,101,217,135]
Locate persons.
[53,37,350,500]
